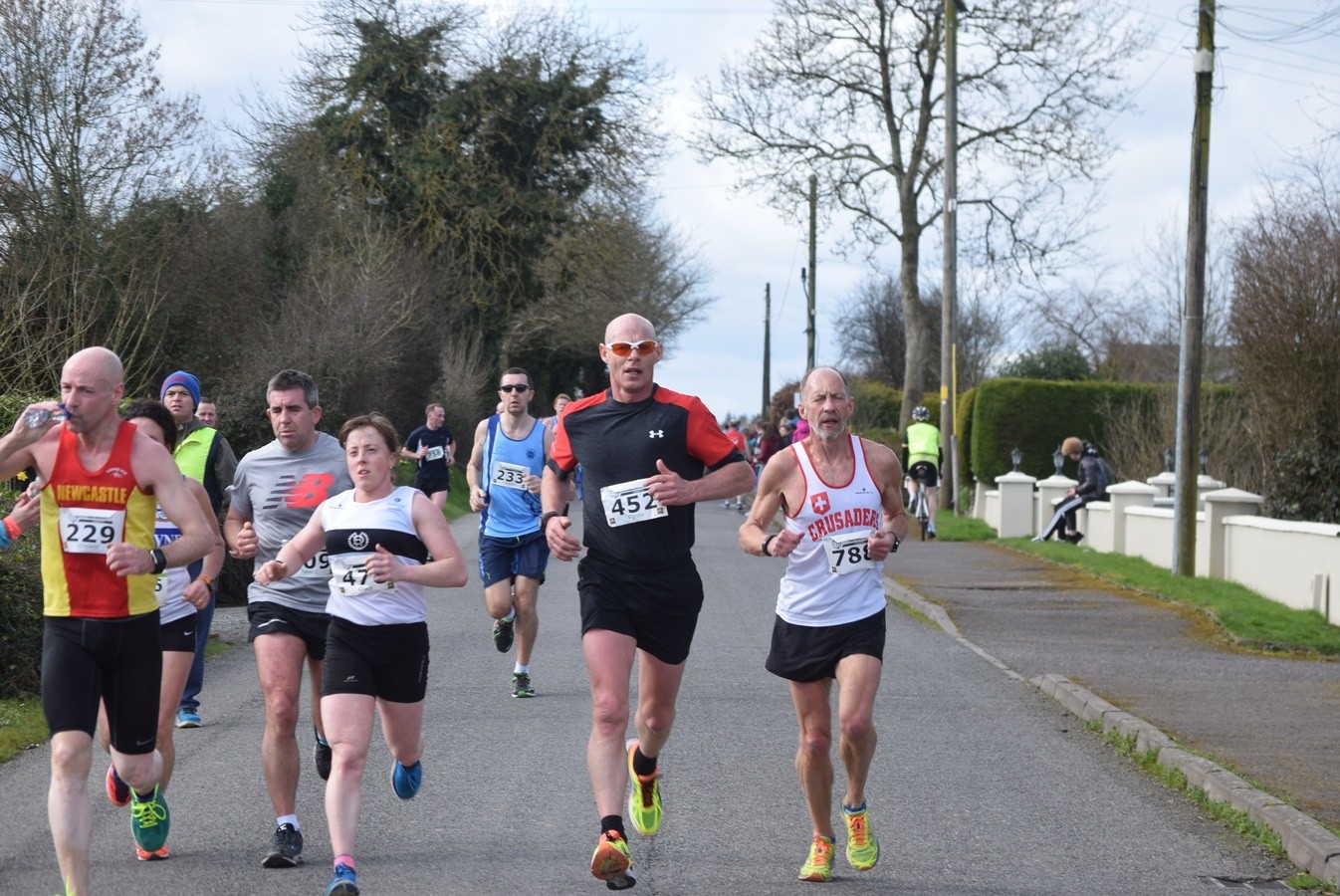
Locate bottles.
[25,403,70,429]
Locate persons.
[539,314,757,891]
[736,365,911,883]
[719,408,810,512]
[254,414,470,896]
[0,346,239,896]
[466,367,583,697]
[1030,437,1116,545]
[400,403,457,562]
[223,369,355,870]
[902,405,945,538]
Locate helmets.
[912,406,930,422]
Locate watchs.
[888,531,900,553]
[196,575,215,596]
[149,547,166,576]
[537,510,560,532]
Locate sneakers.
[799,833,836,881]
[841,796,881,871]
[625,737,664,836]
[314,724,333,780]
[590,830,637,890]
[128,783,171,852]
[326,862,360,896]
[135,839,171,861]
[492,592,516,652]
[389,757,423,802]
[512,672,537,698]
[106,760,131,806]
[174,708,203,729]
[262,822,304,867]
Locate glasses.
[499,384,530,393]
[608,340,659,357]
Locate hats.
[160,371,200,414]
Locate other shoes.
[722,501,730,508]
[908,495,918,514]
[736,502,743,509]
[1067,531,1083,544]
[928,526,936,538]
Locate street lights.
[801,175,818,376]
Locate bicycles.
[912,465,934,541]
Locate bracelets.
[275,559,284,564]
[761,535,777,557]
